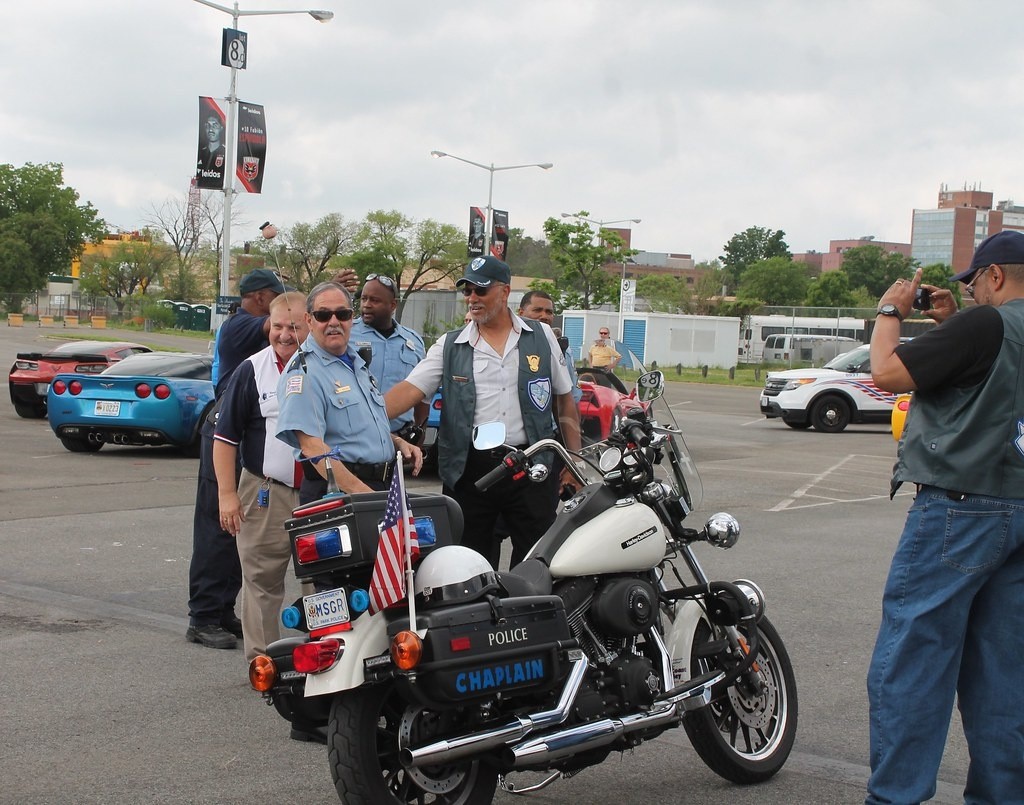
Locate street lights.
[194,0,336,338]
[560,212,642,308]
[430,150,553,257]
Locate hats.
[455,256,511,287]
[240,269,297,294]
[948,231,1024,286]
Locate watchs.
[876,302,903,321]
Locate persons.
[199,110,224,188]
[381,254,584,573]
[861,231,1024,805]
[274,280,424,745]
[470,215,484,255]
[588,340,622,388]
[211,290,312,686]
[187,269,361,650]
[599,327,612,338]
[465,310,473,324]
[518,290,583,510]
[347,269,427,459]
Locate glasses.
[461,283,503,296]
[964,268,987,298]
[312,309,352,322]
[601,332,608,335]
[366,273,396,298]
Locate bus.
[737,314,865,365]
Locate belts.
[342,461,396,482]
[474,445,529,460]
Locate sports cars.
[44,350,216,459]
[6,339,155,420]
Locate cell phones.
[912,288,930,310]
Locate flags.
[368,456,420,616]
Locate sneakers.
[222,619,243,639]
[186,624,238,648]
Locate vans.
[760,334,858,371]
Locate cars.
[759,335,917,443]
[569,365,655,444]
[399,384,442,473]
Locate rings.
[896,281,902,284]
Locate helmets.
[414,546,500,609]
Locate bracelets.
[574,459,586,468]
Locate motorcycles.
[245,368,800,804]
